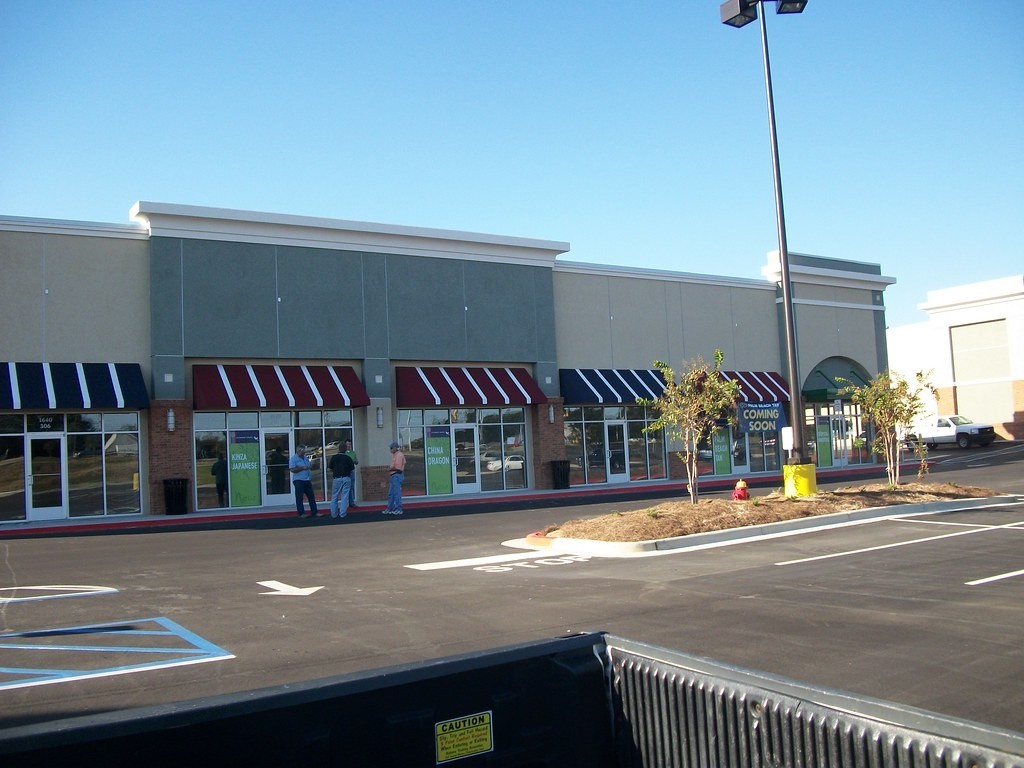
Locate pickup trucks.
[899,415,996,451]
[576,449,624,469]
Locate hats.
[388,441,400,448]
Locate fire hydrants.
[732,479,750,501]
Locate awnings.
[191,363,371,412]
[712,371,790,403]
[395,367,548,409]
[0,363,149,412]
[560,369,687,408]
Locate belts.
[333,475,350,478]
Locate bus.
[455,429,474,450]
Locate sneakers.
[392,509,403,515]
[382,509,392,515]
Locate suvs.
[733,437,766,462]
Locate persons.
[382,442,406,514]
[289,444,323,518]
[344,439,358,509]
[328,442,355,519]
[270,446,289,494]
[211,454,229,508]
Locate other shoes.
[313,513,324,517]
[298,514,308,519]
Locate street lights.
[720,0,812,466]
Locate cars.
[319,442,338,451]
[74,446,101,460]
[469,451,501,465]
[306,451,323,458]
[854,431,867,449]
[305,445,318,451]
[306,455,320,463]
[487,455,524,471]
[699,450,712,460]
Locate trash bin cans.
[551,460,571,490]
[161,478,189,516]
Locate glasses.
[390,447,395,449]
